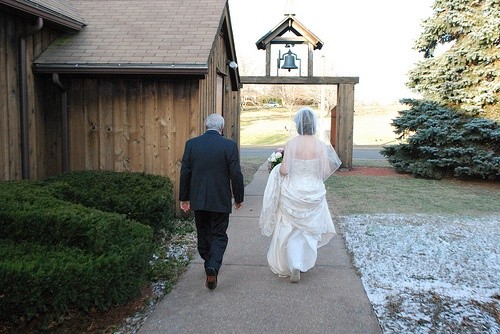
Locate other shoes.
[206,267,217,290]
[290,269,300,282]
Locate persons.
[178,113,244,291]
[259,106,342,282]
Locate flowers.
[267,148,284,174]
